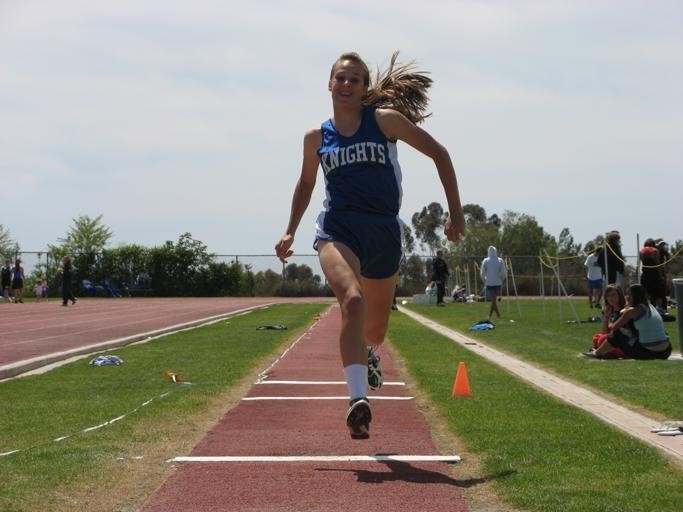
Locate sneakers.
[584,348,601,359]
[346,397,372,439]
[368,344,382,390]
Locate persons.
[0,254,76,307]
[424,245,507,321]
[272,50,466,442]
[575,228,676,362]
